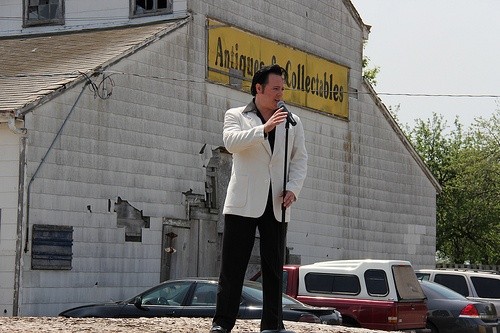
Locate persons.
[209,64,308,333]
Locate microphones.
[277,100,297,126]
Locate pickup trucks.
[250,258,428,330]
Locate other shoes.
[210,325,227,333]
[260,326,295,333]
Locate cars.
[59,268,500,333]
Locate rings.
[290,199,293,203]
